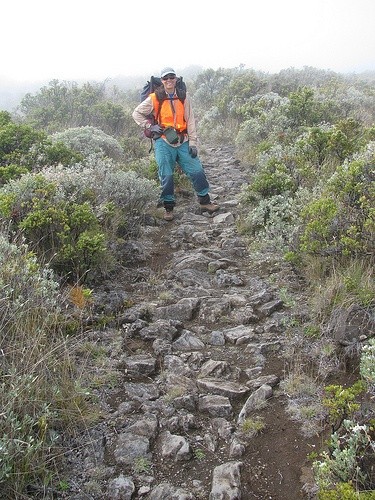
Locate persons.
[132,67,220,221]
